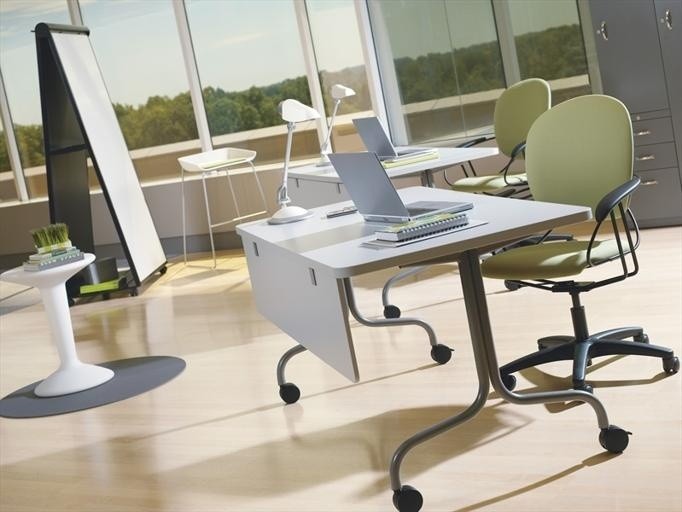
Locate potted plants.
[28,222,72,255]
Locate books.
[79,275,127,295]
[364,218,488,247]
[23,240,84,272]
[375,214,468,241]
[375,155,440,169]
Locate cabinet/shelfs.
[586,1,681,230]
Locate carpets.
[0,353,187,420]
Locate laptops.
[328,152,473,222]
[352,117,431,158]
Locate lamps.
[316,83,356,166]
[266,98,322,225]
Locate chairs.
[443,77,579,290]
[480,93,680,403]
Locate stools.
[176,146,271,270]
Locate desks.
[280,145,502,320]
[0,253,115,399]
[233,187,632,512]
